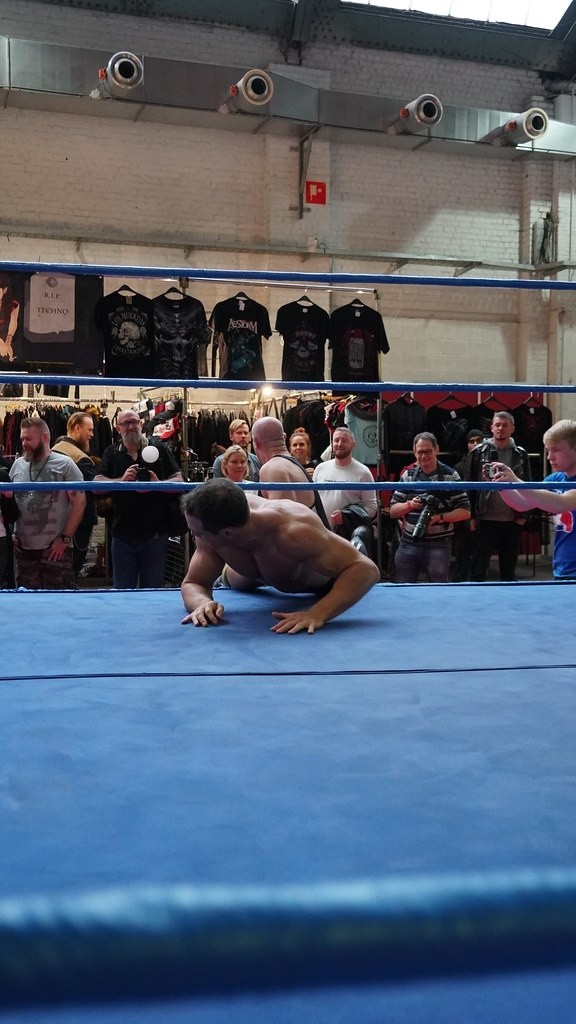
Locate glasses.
[116,420,141,427]
[469,439,482,444]
[415,447,434,455]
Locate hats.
[466,429,486,443]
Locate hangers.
[0,388,390,416]
[479,391,511,412]
[162,276,188,300]
[347,288,367,309]
[396,388,415,405]
[290,284,316,306]
[230,280,255,305]
[116,277,136,296]
[521,391,543,409]
[432,391,470,408]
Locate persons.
[452,428,485,583]
[252,417,332,532]
[92,409,184,588]
[212,418,262,482]
[390,432,471,583]
[483,419,576,581]
[9,417,87,590]
[313,426,378,539]
[463,412,532,582]
[221,445,258,495]
[290,427,316,477]
[50,412,98,578]
[0,447,19,590]
[180,478,381,635]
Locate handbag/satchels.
[155,441,194,536]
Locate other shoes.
[352,524,372,559]
[213,572,225,588]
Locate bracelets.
[407,501,414,511]
[439,514,443,523]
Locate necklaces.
[29,455,50,496]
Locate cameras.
[134,466,151,481]
[412,495,439,542]
[485,463,501,479]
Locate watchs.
[61,534,71,544]
